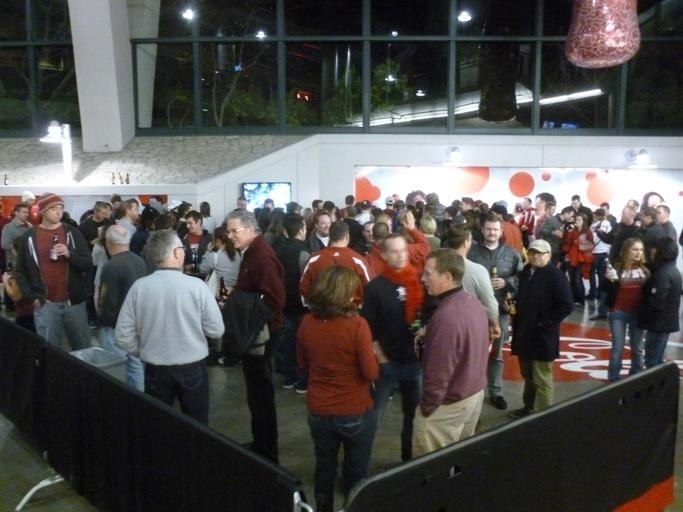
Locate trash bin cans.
[69,346,128,383]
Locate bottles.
[218,276,228,301]
[566,224,576,230]
[490,265,498,291]
[412,310,423,338]
[604,258,619,284]
[191,249,198,267]
[416,323,426,361]
[49,235,59,263]
[506,291,517,316]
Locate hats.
[38,193,64,213]
[592,210,604,216]
[527,239,551,252]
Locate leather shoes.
[591,315,607,320]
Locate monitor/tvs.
[242,181,292,216]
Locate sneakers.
[492,396,528,418]
[284,378,307,393]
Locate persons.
[1,188,285,465]
[412,248,489,459]
[277,190,683,416]
[362,232,442,466]
[292,264,382,512]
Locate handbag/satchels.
[205,270,220,298]
